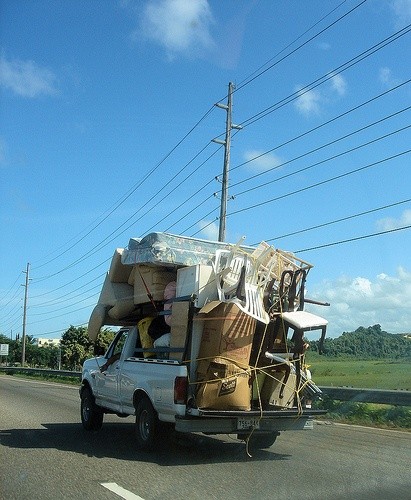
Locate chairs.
[212,236,271,325]
[267,271,328,359]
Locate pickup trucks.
[79,323,329,460]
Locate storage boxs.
[263,372,299,409]
[195,359,252,411]
[193,300,257,379]
